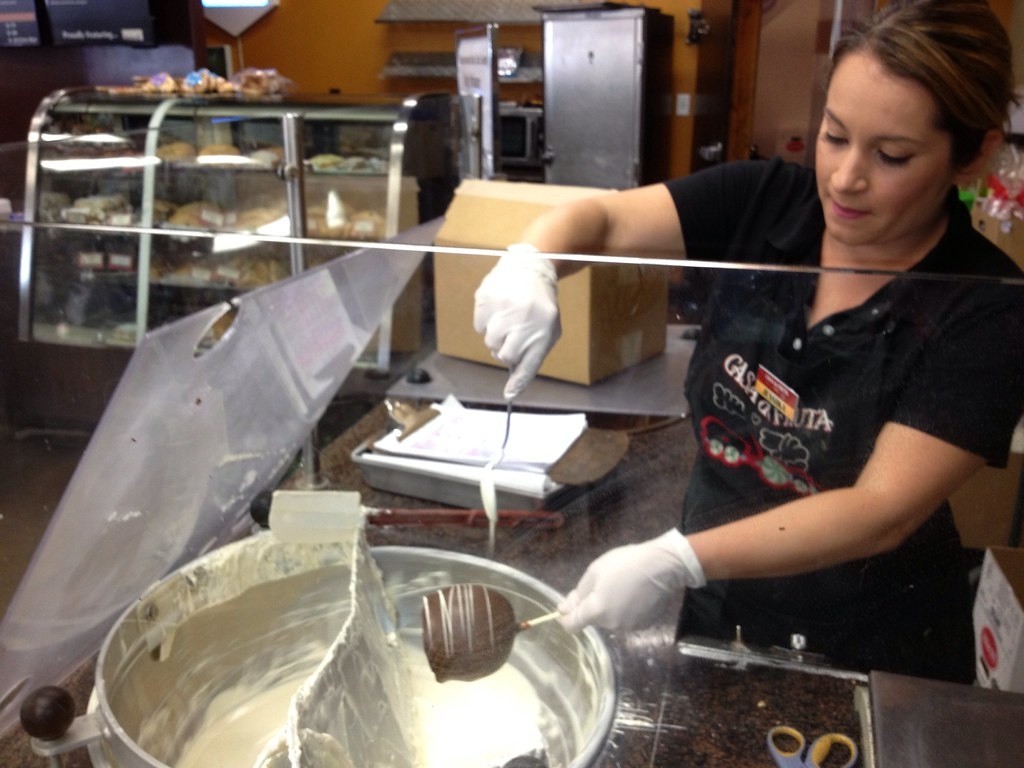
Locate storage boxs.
[434,177,668,389]
[952,452,1023,698]
[970,200,1024,270]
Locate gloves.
[555,527,707,634]
[473,243,562,403]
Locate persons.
[473,0,1024,690]
